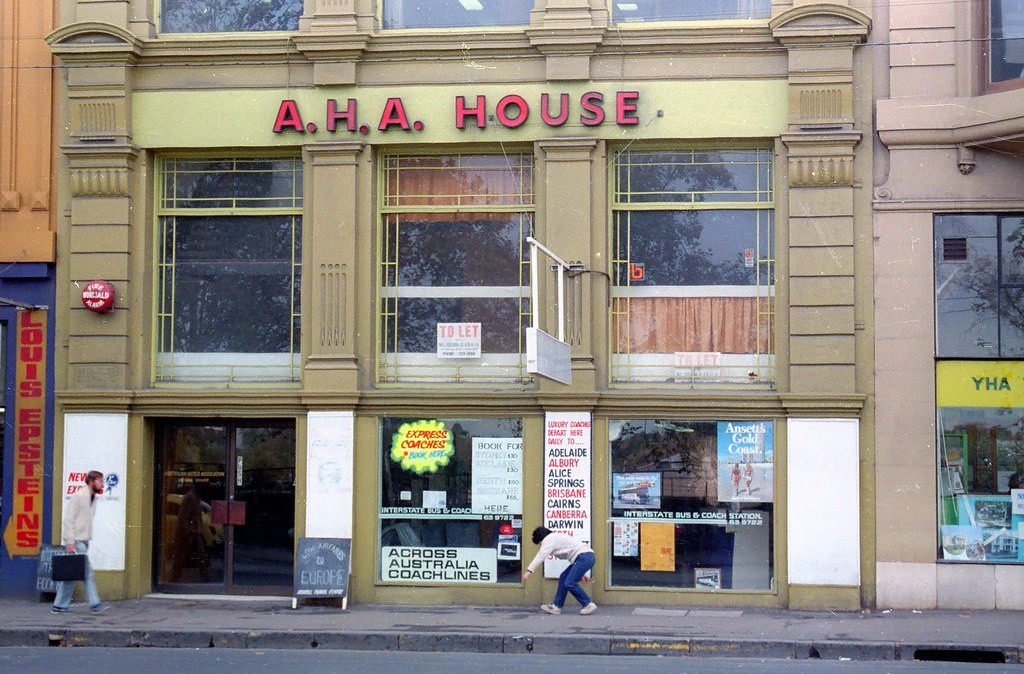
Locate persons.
[731,462,753,496]
[50,470,110,615]
[170,482,213,583]
[521,527,599,614]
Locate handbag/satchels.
[51,549,87,581]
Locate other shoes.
[88,603,111,615]
[51,606,71,614]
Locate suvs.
[631,496,720,562]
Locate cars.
[166,494,224,545]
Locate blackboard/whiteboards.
[35,544,67,593]
[293,537,351,598]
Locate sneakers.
[540,604,561,614]
[579,602,598,615]
